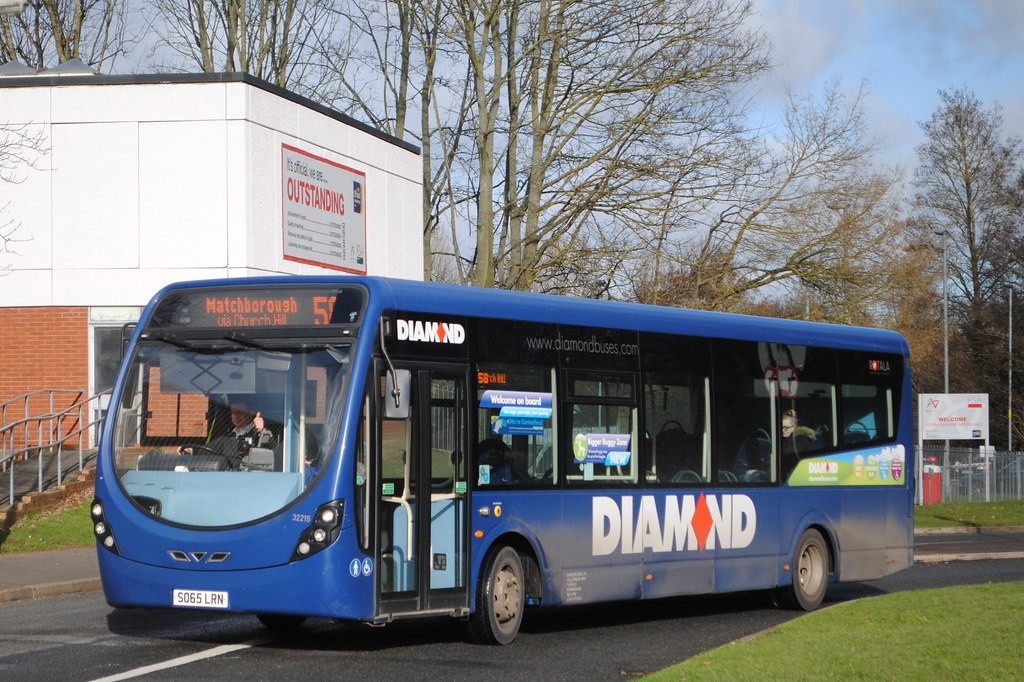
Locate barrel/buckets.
[924,466,941,505]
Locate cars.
[950,455,1024,499]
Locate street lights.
[933,229,951,502]
[1002,282,1015,454]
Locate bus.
[91,277,917,644]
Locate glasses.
[783,425,793,431]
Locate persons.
[742,408,818,484]
[176,400,277,470]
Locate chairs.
[622,420,871,483]
[543,467,568,484]
